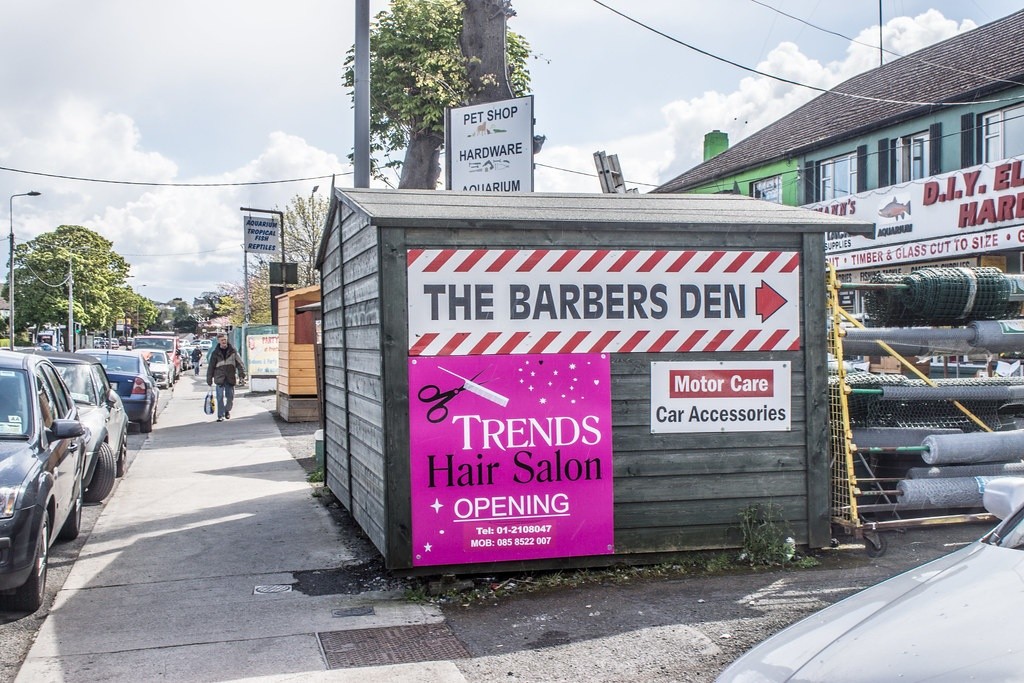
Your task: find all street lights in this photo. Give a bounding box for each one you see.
[135,282,146,336]
[9,190,43,350]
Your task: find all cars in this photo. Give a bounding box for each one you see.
[95,335,133,349]
[178,337,213,371]
[11,350,128,504]
[131,349,177,390]
[0,349,87,614]
[75,346,160,434]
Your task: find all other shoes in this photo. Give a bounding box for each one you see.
[225,411,230,419]
[217,418,223,422]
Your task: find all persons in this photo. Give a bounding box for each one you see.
[192,345,202,375]
[206,332,246,422]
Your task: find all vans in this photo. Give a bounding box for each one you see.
[131,335,182,384]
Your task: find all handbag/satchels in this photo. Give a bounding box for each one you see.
[204,384,216,415]
